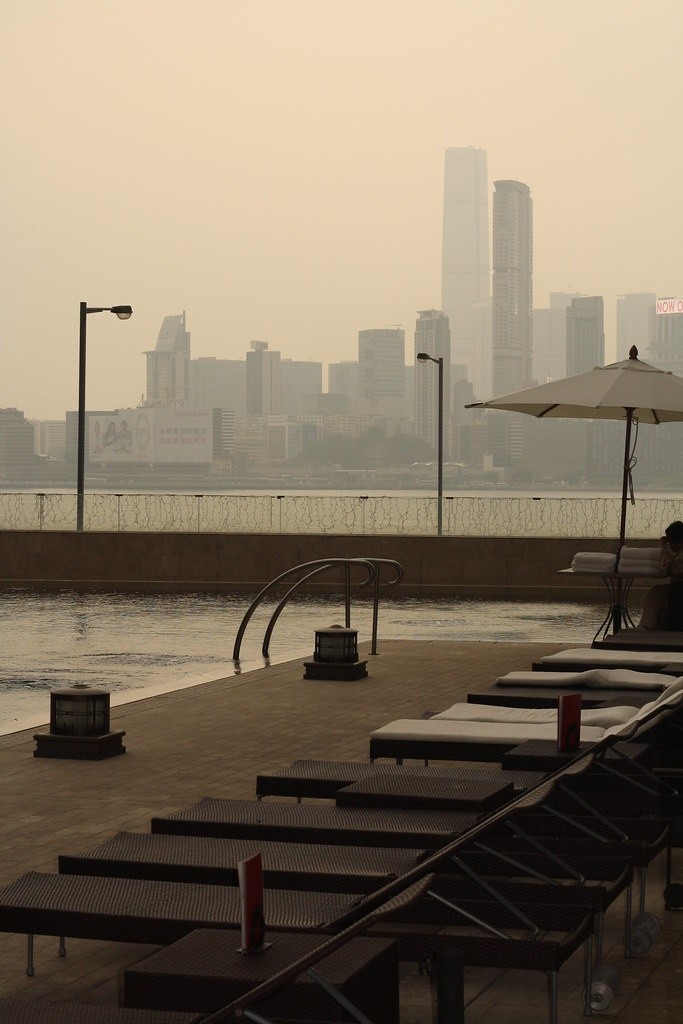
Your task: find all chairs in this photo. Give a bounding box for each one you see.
[420,676,683,729]
[0,780,594,976]
[0,871,434,1024]
[465,669,681,707]
[533,648,683,675]
[59,755,614,992]
[152,720,651,963]
[556,567,666,644]
[256,692,683,911]
[369,690,681,769]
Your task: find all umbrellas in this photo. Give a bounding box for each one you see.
[465,358,683,552]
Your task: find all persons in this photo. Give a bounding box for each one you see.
[640,521,683,629]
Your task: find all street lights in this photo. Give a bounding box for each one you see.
[417,352,442,537]
[77,302,133,532]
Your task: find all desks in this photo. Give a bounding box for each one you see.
[122,926,400,1024]
[660,662,683,675]
[502,739,606,783]
[595,696,657,711]
[336,771,514,813]
